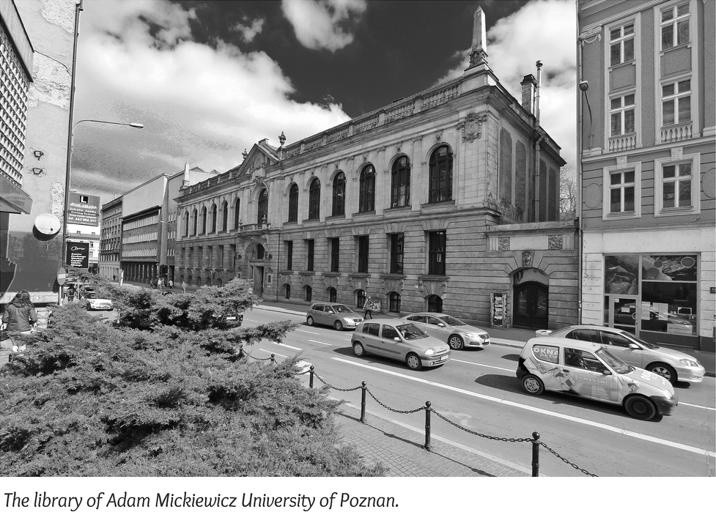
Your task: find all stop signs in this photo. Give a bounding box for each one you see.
[57,268,66,286]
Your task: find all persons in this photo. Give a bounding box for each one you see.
[362,296,373,320]
[157,276,185,294]
[0,288,39,359]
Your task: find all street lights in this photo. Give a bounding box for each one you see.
[59,119,142,267]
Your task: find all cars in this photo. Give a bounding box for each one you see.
[537,324,706,383]
[64,278,114,311]
[408,315,489,350]
[351,319,450,370]
[604,304,692,332]
[517,337,676,420]
[306,303,365,331]
